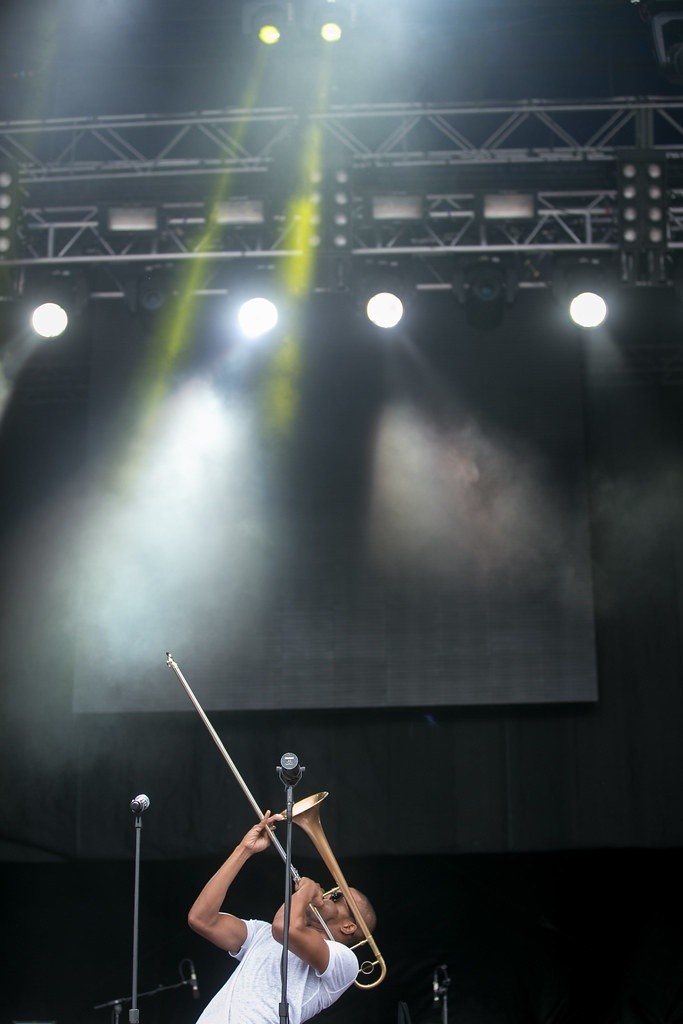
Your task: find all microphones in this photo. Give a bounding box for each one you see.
[431,974,441,1012]
[283,754,298,787]
[132,793,150,816]
[189,970,200,995]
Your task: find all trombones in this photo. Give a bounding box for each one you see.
[162,650,389,991]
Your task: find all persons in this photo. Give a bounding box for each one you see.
[186,809,376,1023]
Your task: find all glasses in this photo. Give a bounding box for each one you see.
[329,892,355,922]
